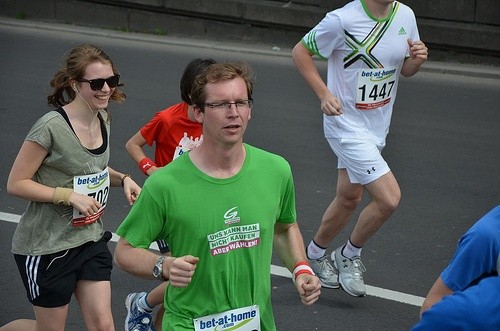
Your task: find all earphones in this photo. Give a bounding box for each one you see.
[72,83,76,86]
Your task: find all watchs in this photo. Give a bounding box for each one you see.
[153,256,169,281]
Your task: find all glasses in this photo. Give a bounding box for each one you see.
[73,73,120,92]
[205,98,253,115]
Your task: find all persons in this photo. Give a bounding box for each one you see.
[291,0,428,296]
[420,204,500,331]
[115,62,321,331]
[0,44,142,331]
[411,277,500,331]
[125,58,217,331]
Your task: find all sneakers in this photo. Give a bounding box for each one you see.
[305,247,341,289]
[331,244,367,298]
[124,291,152,331]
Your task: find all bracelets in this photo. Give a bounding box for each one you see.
[121,174,132,188]
[293,261,314,280]
[137,157,157,175]
[53,187,73,205]
